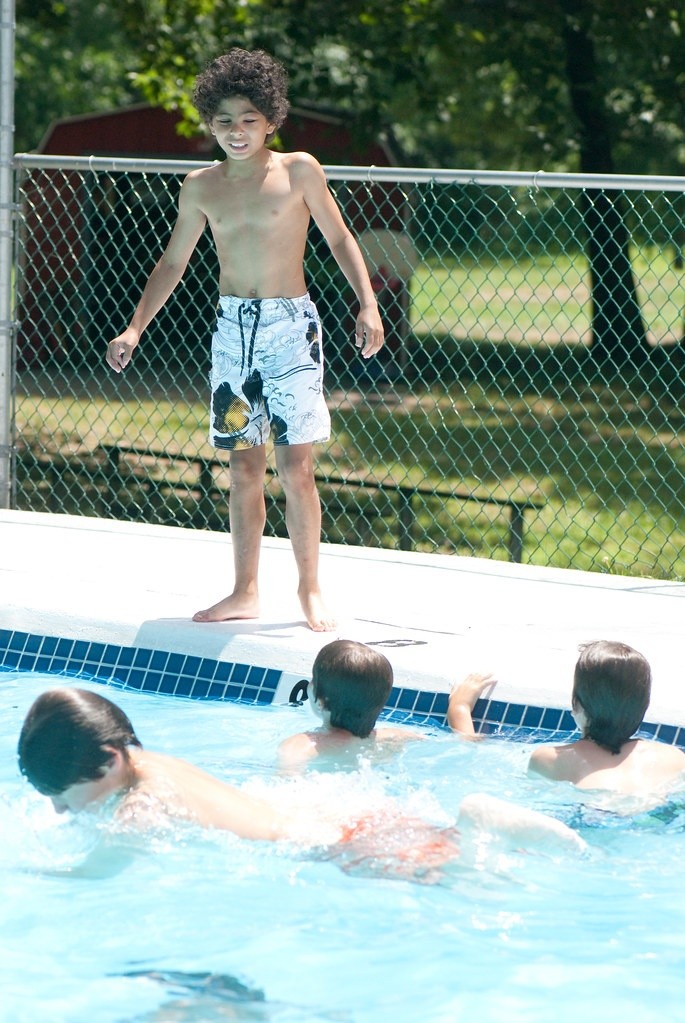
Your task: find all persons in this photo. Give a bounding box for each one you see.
[278,639,498,758]
[105,48,385,632]
[528,641,685,789]
[17,687,293,840]
